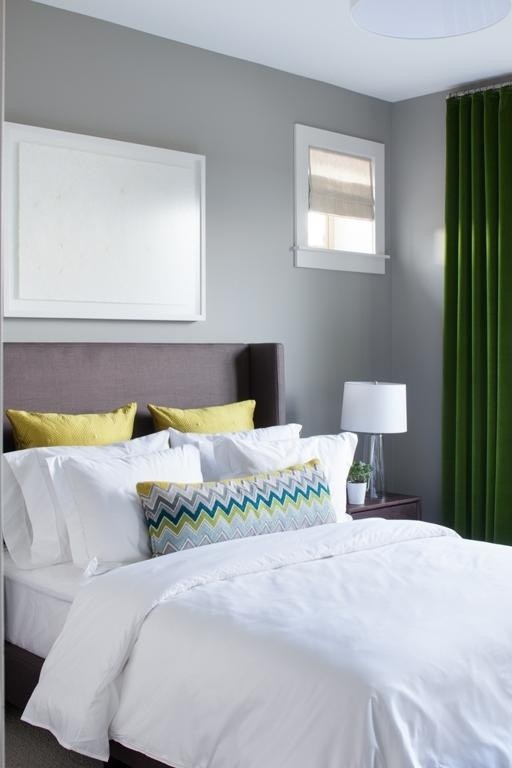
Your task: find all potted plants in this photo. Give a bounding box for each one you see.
[346,461,375,504]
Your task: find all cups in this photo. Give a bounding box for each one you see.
[347,481,367,504]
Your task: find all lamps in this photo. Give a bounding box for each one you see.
[349,1,512,39]
[339,379,409,503]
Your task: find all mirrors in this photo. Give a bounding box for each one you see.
[288,121,391,276]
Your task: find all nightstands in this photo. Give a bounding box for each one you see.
[345,491,421,520]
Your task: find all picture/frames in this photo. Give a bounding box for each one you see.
[2,122,206,321]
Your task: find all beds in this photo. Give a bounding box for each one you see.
[2,341,511,768]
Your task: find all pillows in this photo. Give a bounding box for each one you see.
[46,441,209,576]
[168,422,302,482]
[137,457,336,557]
[4,400,137,449]
[211,430,359,523]
[2,426,172,571]
[146,399,258,434]
